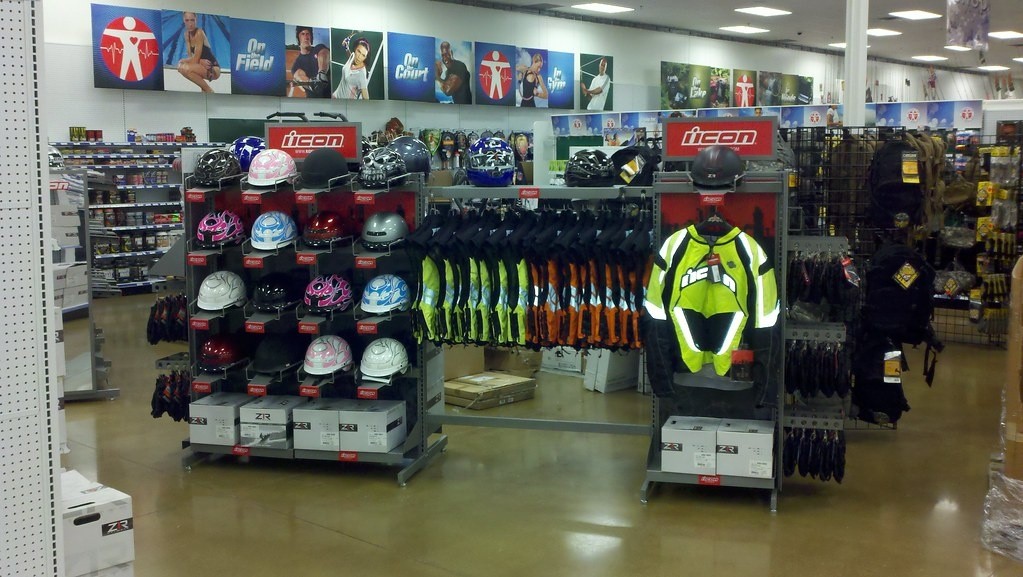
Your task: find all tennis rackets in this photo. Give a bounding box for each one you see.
[341,30,363,47]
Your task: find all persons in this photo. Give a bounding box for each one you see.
[332,30,370,100]
[581,57,610,110]
[826,106,842,126]
[928,66,937,101]
[664,64,688,109]
[605,133,620,145]
[178,12,220,92]
[658,111,696,119]
[516,53,548,107]
[755,108,762,117]
[435,41,472,104]
[291,26,330,98]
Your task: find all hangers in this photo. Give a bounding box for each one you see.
[429,192,652,257]
[695,206,733,232]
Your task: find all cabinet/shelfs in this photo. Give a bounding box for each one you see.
[47,142,448,483]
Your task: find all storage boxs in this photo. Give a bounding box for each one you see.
[661,415,777,479]
[443,371,537,410]
[49,200,406,577]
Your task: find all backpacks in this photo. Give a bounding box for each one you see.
[611,136,660,185]
[844,132,951,423]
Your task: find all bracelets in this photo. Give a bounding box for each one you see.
[535,92,539,96]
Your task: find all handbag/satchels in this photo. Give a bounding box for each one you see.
[932,261,976,293]
[940,222,976,248]
[943,175,977,204]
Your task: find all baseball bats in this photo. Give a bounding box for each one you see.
[285,79,322,88]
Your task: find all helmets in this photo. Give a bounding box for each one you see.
[360,337,408,377]
[564,150,616,187]
[199,333,241,369]
[252,272,299,311]
[357,147,406,189]
[302,210,347,246]
[465,138,515,186]
[362,143,371,156]
[250,210,296,250]
[254,335,301,372]
[693,145,744,185]
[304,335,352,375]
[194,149,241,185]
[197,271,247,310]
[360,274,412,314]
[229,135,265,171]
[386,137,431,178]
[361,212,408,248]
[298,148,348,186]
[196,209,245,246]
[247,149,297,186]
[304,275,353,312]
